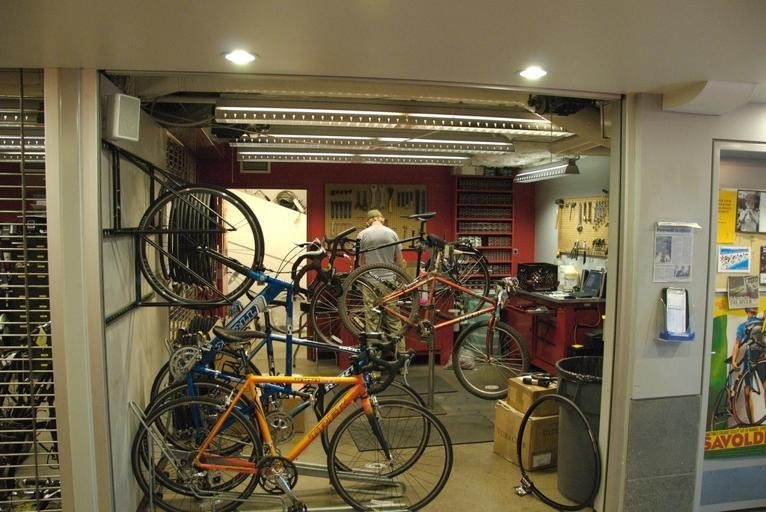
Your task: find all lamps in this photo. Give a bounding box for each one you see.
[566,156,580,174]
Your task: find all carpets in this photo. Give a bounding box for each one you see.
[328,375,493,451]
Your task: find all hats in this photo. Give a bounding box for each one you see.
[368,209,382,220]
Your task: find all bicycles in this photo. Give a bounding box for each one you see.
[708,322,766,432]
[0,314,64,512]
[337,232,531,400]
[134,182,490,511]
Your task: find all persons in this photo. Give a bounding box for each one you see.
[352,207,410,385]
[738,193,760,233]
[731,307,766,425]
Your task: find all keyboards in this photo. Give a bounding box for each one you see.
[569,293,592,298]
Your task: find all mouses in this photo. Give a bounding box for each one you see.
[565,296,576,299]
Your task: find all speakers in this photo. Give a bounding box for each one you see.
[108,94,141,143]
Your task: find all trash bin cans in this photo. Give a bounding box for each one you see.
[555,356,603,504]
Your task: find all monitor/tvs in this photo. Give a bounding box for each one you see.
[582,270,607,296]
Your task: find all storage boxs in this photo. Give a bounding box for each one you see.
[493,371,559,472]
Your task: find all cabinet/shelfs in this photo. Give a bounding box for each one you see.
[454,176,515,324]
[504,292,605,376]
